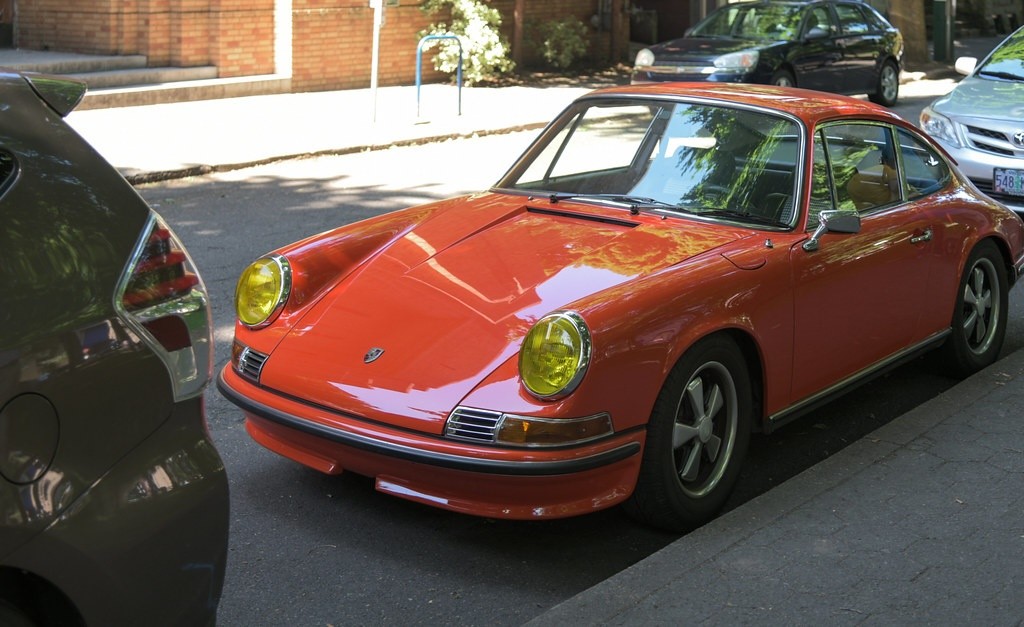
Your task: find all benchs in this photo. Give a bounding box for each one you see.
[730,156,938,207]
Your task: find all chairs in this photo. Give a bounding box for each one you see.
[664,146,860,228]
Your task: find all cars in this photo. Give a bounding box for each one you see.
[0,70,229,626]
[630,0,906,107]
[220,78,1024,526]
[918,25,1024,214]
